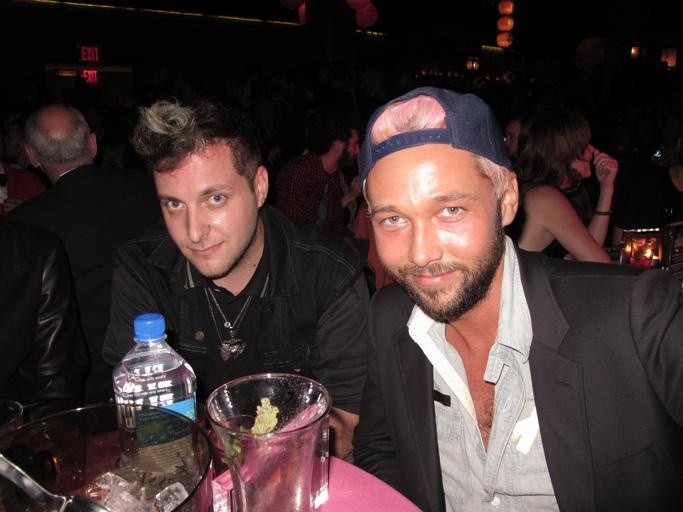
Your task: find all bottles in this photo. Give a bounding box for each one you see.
[109,312,198,430]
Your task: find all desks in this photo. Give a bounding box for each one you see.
[0,431,423,512]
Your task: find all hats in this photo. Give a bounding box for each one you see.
[358,86,522,184]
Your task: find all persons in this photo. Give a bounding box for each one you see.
[1,60,683,512]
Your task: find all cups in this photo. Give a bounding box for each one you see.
[203,372,331,512]
